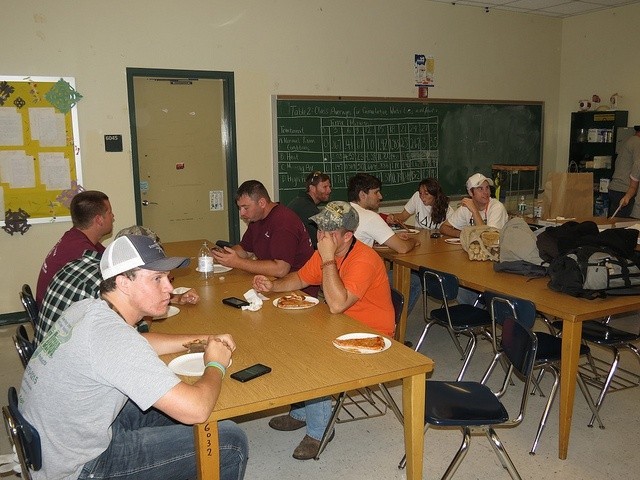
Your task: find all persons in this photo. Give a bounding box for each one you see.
[11,235,249,480]
[607,125,640,219]
[286,172,332,251]
[35,191,115,317]
[611,158,640,219]
[252,200,396,460]
[33,225,199,351]
[440,173,509,310]
[209,180,318,298]
[345,173,422,348]
[386,177,454,229]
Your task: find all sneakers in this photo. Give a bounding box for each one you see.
[269,415,306,431]
[293,429,335,460]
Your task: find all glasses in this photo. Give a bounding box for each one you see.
[312,172,321,185]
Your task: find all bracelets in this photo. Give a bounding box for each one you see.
[321,259,337,269]
[205,362,225,380]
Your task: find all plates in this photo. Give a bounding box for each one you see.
[332,332,393,355]
[195,263,234,274]
[393,228,421,235]
[443,238,462,245]
[151,304,181,320]
[167,351,233,377]
[273,294,320,310]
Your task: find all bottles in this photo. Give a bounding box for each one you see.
[517,195,528,222]
[197,240,215,282]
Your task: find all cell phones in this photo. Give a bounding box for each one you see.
[230,363,271,383]
[212,247,222,253]
[430,233,440,238]
[222,297,249,309]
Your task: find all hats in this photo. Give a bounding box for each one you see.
[114,225,160,242]
[466,173,494,190]
[308,201,359,231]
[100,235,190,281]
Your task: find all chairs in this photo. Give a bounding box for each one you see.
[2,385,43,480]
[18,283,37,327]
[481,289,605,456]
[398,315,540,480]
[530,316,639,429]
[414,265,515,389]
[11,323,33,369]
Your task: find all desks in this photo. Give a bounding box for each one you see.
[394,248,640,461]
[506,204,639,250]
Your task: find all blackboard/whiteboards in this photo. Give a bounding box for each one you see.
[270,94,545,212]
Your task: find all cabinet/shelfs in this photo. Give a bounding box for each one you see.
[567,110,628,214]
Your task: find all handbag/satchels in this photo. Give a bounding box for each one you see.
[536,221,600,264]
[600,227,639,250]
[494,261,548,277]
[541,160,594,222]
[460,226,500,262]
[548,247,640,300]
[500,218,561,268]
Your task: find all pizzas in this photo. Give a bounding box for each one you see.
[332,335,386,353]
[276,292,317,309]
[182,338,208,351]
